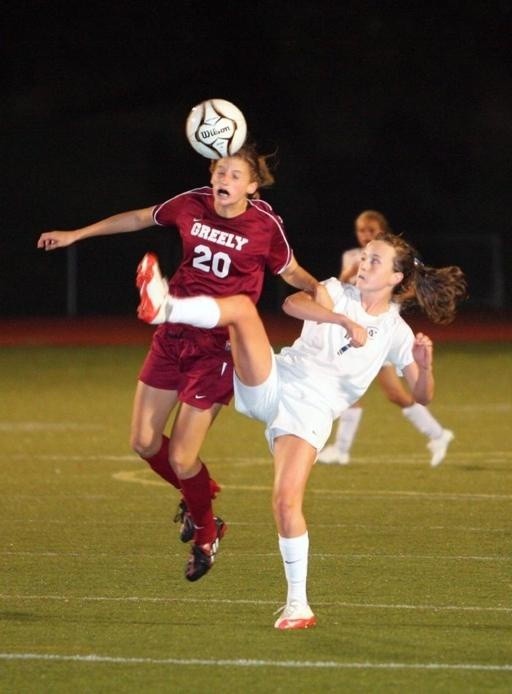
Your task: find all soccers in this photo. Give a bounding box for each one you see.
[186,96,249,162]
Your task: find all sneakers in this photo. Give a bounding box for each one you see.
[273,603,317,631]
[426,429,455,467]
[179,478,228,582]
[135,251,171,326]
[317,444,350,465]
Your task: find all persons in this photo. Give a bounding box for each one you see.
[320,210,455,468]
[134,233,467,632]
[38,146,319,582]
